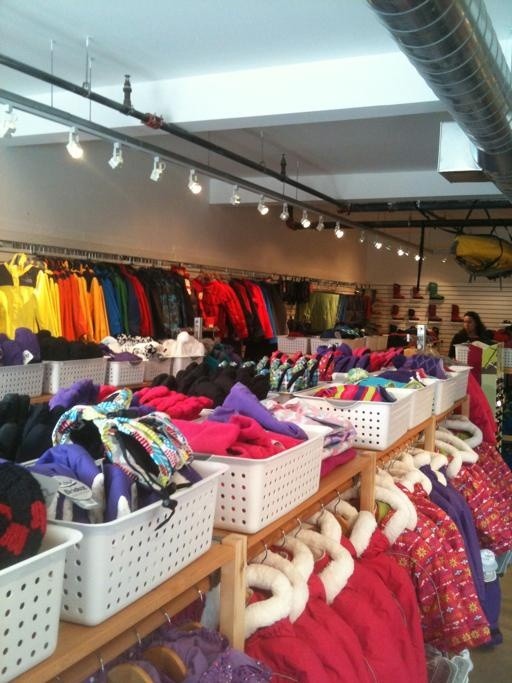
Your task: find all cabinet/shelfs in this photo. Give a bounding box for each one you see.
[481,372,504,453]
[389,296,466,347]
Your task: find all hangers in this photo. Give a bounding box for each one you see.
[250,487,353,575]
[374,434,426,477]
[434,407,457,434]
[12,243,168,282]
[52,584,207,683]
[195,263,256,285]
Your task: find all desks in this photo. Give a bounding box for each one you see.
[435,394,471,435]
[211,453,374,565]
[28,380,152,405]
[1,530,244,683]
[354,416,435,470]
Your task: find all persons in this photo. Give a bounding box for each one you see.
[448,311,493,359]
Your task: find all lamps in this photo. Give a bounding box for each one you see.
[2,97,430,262]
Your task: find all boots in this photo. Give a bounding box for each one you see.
[412,287,423,299]
[428,304,442,321]
[451,304,464,322]
[429,283,444,300]
[408,309,420,320]
[391,305,405,319]
[393,283,405,299]
